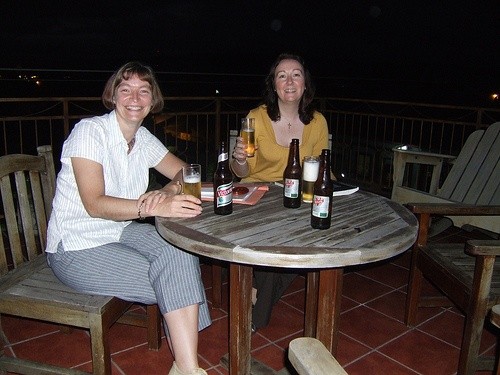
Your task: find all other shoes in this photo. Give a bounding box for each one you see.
[168,362,208,375]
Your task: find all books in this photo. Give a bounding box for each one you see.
[275,175,359,196]
[197,184,257,202]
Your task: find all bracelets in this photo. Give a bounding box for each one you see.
[138,200,145,220]
[171,180,183,195]
[236,159,247,166]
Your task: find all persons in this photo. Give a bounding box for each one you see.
[231,53,329,334]
[46,65,214,375]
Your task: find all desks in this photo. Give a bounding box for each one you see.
[155,182,419,375]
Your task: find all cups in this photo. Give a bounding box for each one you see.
[303,156,319,203]
[241,118,255,158]
[182,164,202,205]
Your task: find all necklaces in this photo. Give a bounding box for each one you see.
[281,115,296,128]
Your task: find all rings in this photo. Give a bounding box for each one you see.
[157,193,162,197]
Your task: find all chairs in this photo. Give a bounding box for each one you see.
[0,145,162,375]
[392,122,500,375]
[211,130,333,338]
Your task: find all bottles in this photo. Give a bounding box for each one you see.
[282,138,302,209]
[214,140,233,216]
[312,148,333,230]
